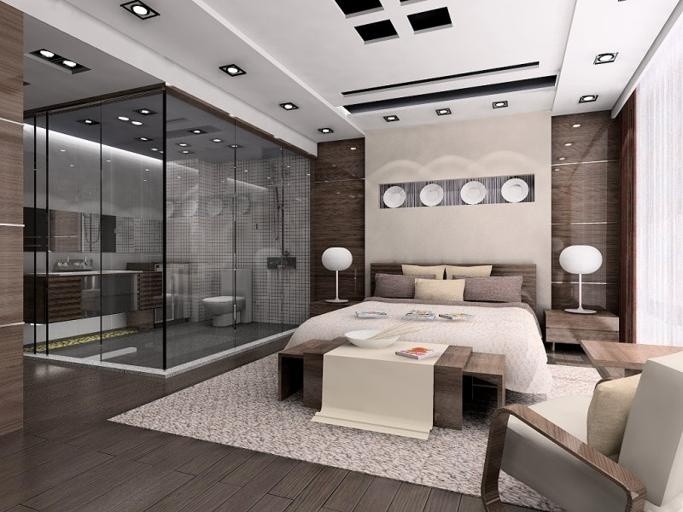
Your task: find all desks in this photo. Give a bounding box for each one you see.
[579,340,682,379]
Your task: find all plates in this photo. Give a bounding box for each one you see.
[346,329,399,348]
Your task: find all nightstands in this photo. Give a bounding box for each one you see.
[543,305,620,352]
[309,302,358,317]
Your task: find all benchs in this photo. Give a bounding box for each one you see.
[278,337,506,430]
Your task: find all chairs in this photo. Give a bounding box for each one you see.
[481,350,682,511]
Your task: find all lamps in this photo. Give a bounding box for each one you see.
[559,244,603,314]
[322,246,354,302]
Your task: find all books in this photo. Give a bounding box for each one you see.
[439,313,472,318]
[403,310,436,320]
[356,310,387,319]
[395,347,434,359]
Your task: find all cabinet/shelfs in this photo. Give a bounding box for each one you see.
[23,206,163,254]
[127,262,191,332]
[22,271,161,324]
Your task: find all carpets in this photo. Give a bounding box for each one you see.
[23,326,141,353]
[106,350,604,511]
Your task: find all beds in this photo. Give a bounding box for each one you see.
[282,263,552,395]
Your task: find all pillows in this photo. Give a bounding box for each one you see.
[586,372,643,457]
[415,278,465,302]
[401,264,446,279]
[465,277,524,302]
[446,265,493,281]
[375,273,435,299]
